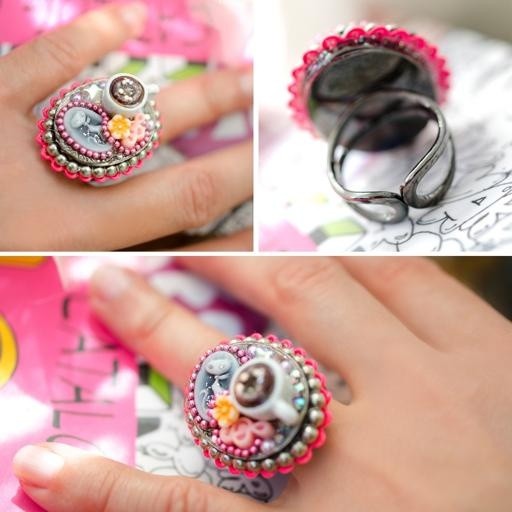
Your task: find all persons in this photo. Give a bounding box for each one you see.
[0,0,253,251]
[10,255,512,511]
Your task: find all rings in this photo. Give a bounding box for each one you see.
[35,70,166,185]
[285,17,457,224]
[183,331,333,478]
